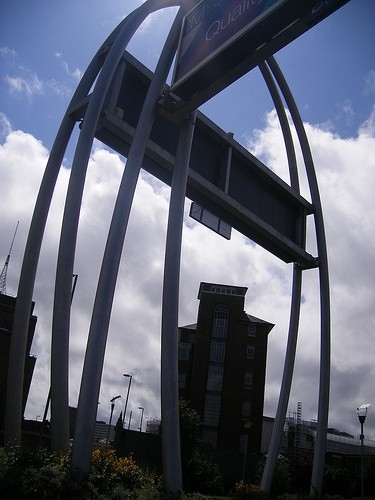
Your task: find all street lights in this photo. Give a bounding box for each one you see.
[104,395,122,454]
[123,373,132,429]
[138,406,145,432]
